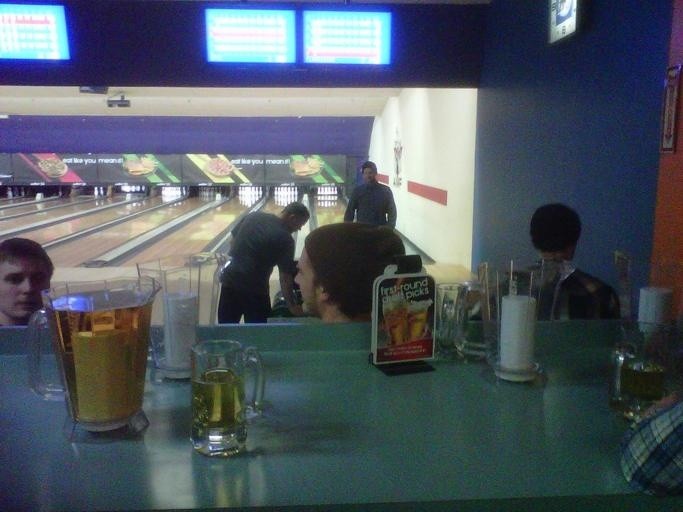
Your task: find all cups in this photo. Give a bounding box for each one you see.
[185,339,266,460]
[435,284,467,360]
[621,319,669,421]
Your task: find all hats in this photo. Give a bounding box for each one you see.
[361,162,377,171]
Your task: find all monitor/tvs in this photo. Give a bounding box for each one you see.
[1,0,76,70]
[299,7,397,76]
[199,3,299,83]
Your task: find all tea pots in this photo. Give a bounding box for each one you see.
[26,252,232,445]
[453,258,577,384]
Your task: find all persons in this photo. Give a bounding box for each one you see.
[217,201,310,324]
[511,201,621,320]
[343,161,398,231]
[1,238,54,326]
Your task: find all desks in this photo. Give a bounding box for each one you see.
[1,317,683,511]
